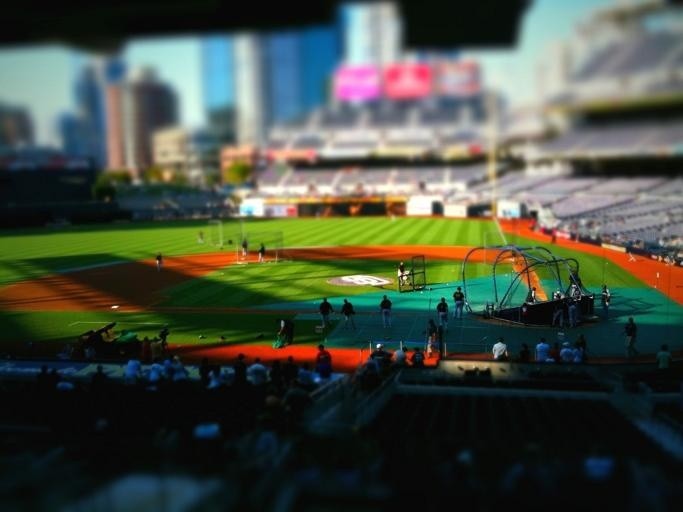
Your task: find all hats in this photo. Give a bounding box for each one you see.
[376,344,382,349]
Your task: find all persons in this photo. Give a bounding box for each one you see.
[1,169,683,512]
